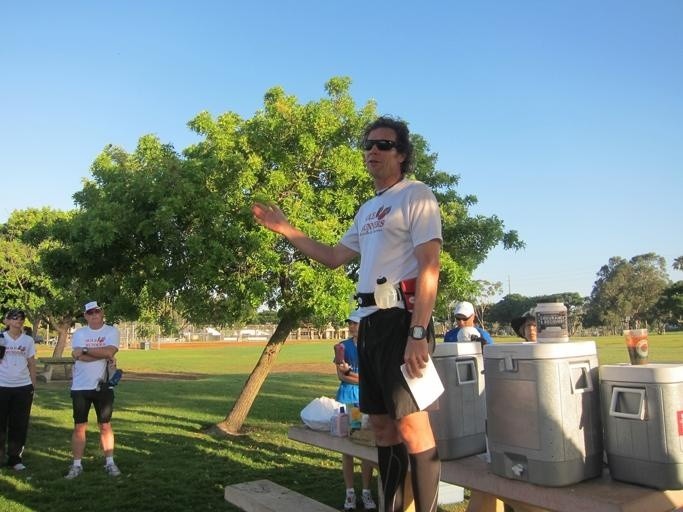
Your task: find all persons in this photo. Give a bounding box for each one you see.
[0,308,40,474]
[333,313,378,512]
[252,117,445,512]
[511,307,538,343]
[0,308,33,336]
[64,301,123,480]
[442,302,493,353]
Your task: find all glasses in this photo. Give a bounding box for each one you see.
[456,315,473,321]
[8,315,25,320]
[363,140,400,150]
[86,309,102,315]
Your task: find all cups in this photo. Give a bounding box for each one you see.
[623,329,650,366]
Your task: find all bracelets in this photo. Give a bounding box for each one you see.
[344,368,353,375]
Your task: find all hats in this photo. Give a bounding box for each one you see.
[511,311,537,336]
[9,308,24,317]
[84,301,102,313]
[457,327,480,342]
[454,301,474,317]
[344,311,361,323]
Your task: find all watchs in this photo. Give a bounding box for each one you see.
[82,346,88,356]
[408,324,429,341]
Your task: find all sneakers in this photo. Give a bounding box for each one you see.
[361,489,376,509]
[64,465,83,479]
[12,463,26,470]
[344,489,357,510]
[104,464,121,477]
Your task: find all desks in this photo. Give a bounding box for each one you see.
[288,421,681,512]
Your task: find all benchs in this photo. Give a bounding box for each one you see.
[221,479,339,512]
